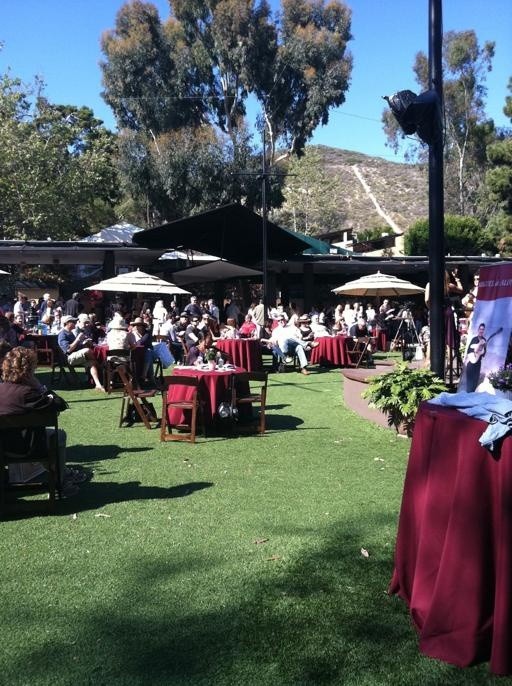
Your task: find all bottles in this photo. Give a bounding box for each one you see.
[130,318,148,325]
[62,315,78,323]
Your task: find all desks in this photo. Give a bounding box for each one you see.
[162,365,249,432]
[387,398,512,676]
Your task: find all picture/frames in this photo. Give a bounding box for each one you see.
[387,398,512,676]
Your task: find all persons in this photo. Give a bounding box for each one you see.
[184,328,229,365]
[0,293,481,382]
[350,320,369,338]
[464,320,489,395]
[0,346,94,488]
[268,314,320,375]
[425,269,464,371]
[462,270,480,310]
[58,315,106,393]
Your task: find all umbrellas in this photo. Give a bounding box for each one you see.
[311,342,319,348]
[301,369,310,375]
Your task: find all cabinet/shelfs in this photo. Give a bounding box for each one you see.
[216,352,224,371]
[197,356,202,368]
[38,321,51,335]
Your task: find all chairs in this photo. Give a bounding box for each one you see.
[159,375,207,444]
[116,363,158,430]
[1,411,63,509]
[238,371,270,432]
[0,324,369,392]
